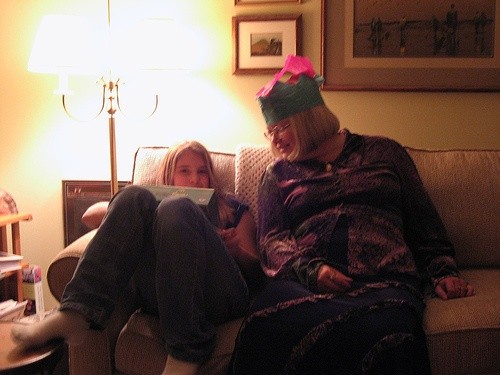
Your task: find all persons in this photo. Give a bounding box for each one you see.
[10,141,259,375]
[225,76,475,375]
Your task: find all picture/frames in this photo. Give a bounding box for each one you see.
[320,0,500,93]
[232,13,304,75]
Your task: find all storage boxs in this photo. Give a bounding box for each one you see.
[24,263,45,317]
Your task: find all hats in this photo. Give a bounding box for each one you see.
[254,53,325,127]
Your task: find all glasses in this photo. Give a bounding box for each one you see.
[264,123,290,139]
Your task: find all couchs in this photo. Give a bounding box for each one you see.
[47,146,500,375]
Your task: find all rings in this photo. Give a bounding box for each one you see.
[330,272,336,280]
[454,285,461,288]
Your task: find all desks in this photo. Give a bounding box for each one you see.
[0,211,33,302]
[0,321,70,375]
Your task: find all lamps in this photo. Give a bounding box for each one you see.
[28,0,160,197]
